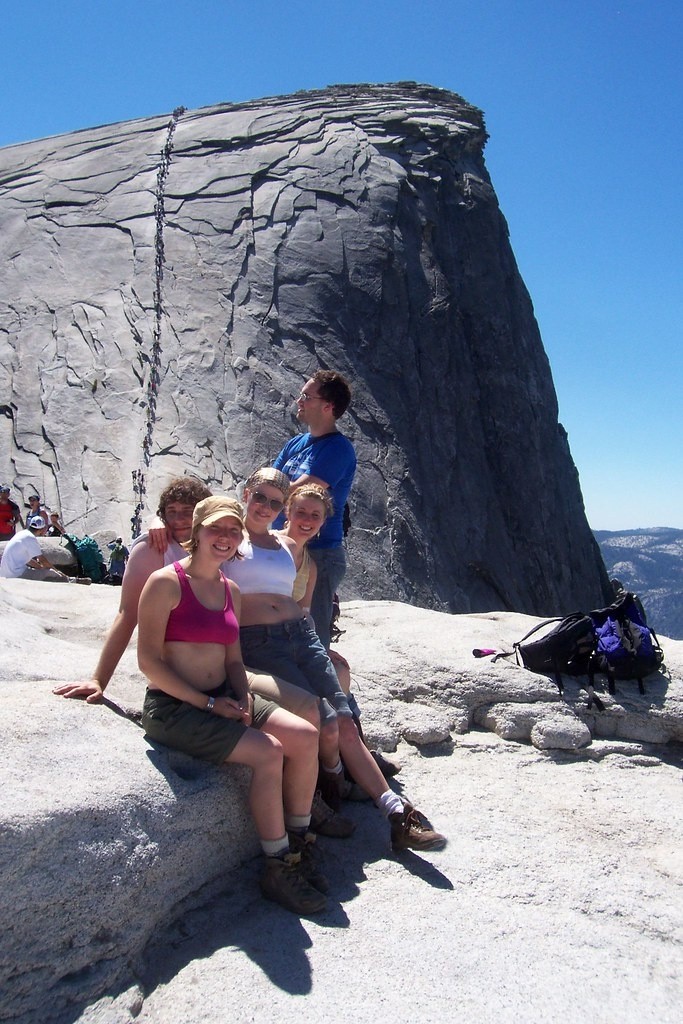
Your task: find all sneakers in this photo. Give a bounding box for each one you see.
[369,747,402,779]
[287,830,329,895]
[387,803,449,855]
[259,850,326,914]
[322,766,372,803]
[311,790,358,839]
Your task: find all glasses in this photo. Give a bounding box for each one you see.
[298,392,324,401]
[248,490,285,514]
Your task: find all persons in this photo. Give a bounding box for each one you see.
[149,468,445,851]
[26,495,49,537]
[136,495,331,915]
[47,513,66,537]
[266,483,401,802]
[0,486,21,541]
[106,538,129,575]
[52,478,355,838]
[273,369,357,651]
[0,516,91,586]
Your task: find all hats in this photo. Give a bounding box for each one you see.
[30,515,45,530]
[115,537,123,543]
[191,495,245,529]
[0,486,10,493]
[29,494,41,502]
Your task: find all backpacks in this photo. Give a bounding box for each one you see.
[111,543,125,561]
[513,611,607,683]
[588,592,664,681]
[68,534,109,582]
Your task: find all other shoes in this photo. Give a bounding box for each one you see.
[76,576,91,585]
